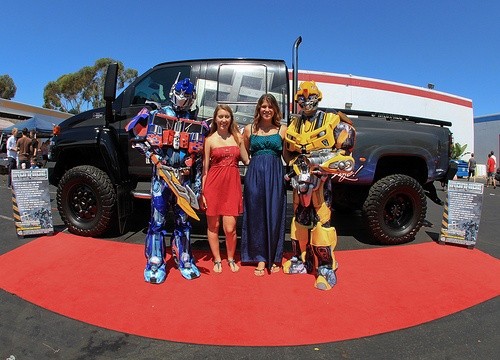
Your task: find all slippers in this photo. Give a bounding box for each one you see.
[228,260,240,272]
[270,264,280,272]
[254,268,265,276]
[213,261,222,273]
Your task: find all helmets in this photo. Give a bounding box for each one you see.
[168,72,197,111]
[294,80,322,111]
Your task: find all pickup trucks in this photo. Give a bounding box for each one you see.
[43,36,458,246]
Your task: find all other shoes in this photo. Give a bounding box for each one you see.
[485,185,489,187]
[178,258,200,280]
[493,186,496,189]
[144,261,166,285]
[7,187,12,189]
[282,256,313,274]
[312,265,337,290]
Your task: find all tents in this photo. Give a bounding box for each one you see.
[0,114,66,156]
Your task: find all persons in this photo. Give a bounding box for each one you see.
[241,94,288,276]
[199,104,250,272]
[124,71,210,285]
[441,151,497,191]
[281,80,357,290]
[6,127,51,189]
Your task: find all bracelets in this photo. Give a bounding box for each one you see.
[199,193,204,194]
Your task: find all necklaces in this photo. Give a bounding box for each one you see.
[260,126,268,130]
